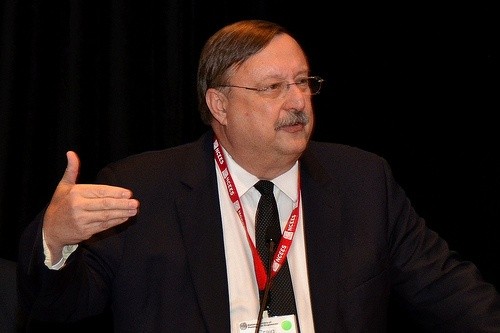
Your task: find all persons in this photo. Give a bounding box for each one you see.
[0,20,500,333]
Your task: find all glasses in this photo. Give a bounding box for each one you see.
[216,77,324,101]
[253,179,301,333]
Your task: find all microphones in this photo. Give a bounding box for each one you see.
[255,225,279,333]
